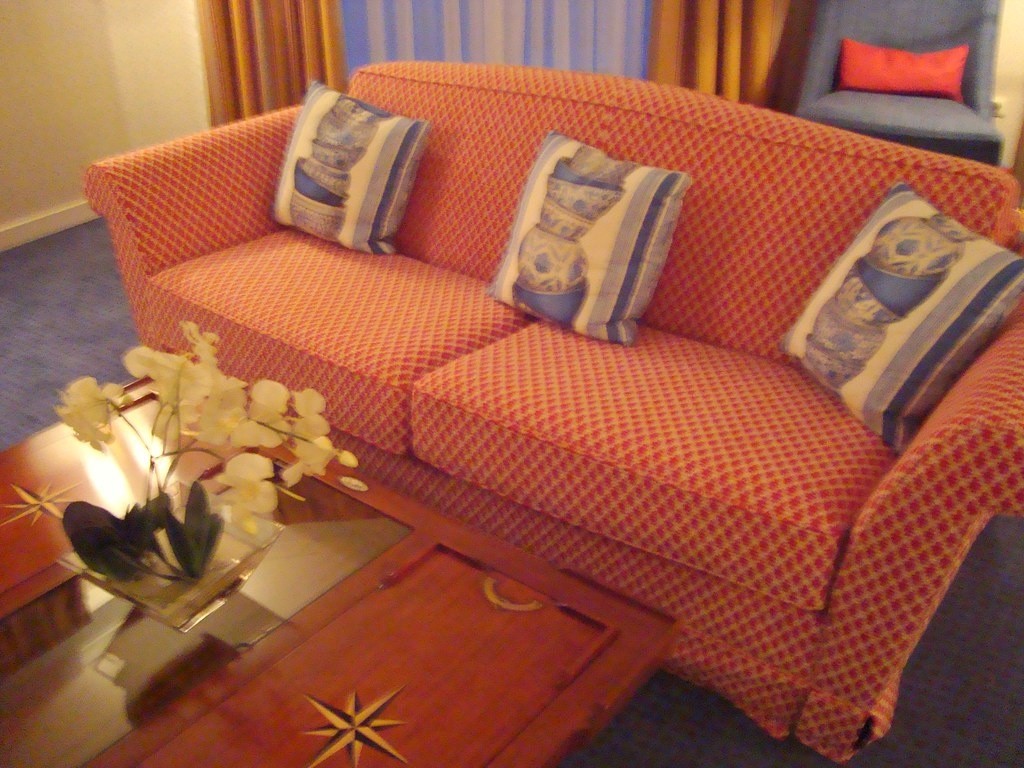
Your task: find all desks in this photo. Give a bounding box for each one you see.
[0,357,680,768]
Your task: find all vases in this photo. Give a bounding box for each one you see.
[53,478,287,634]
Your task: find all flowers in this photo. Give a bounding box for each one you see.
[53,321,359,586]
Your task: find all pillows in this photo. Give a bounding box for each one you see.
[780,183,1024,454]
[837,37,969,106]
[486,127,695,347]
[270,78,433,254]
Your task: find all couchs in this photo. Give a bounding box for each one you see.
[794,0,1004,167]
[82,61,1024,762]
